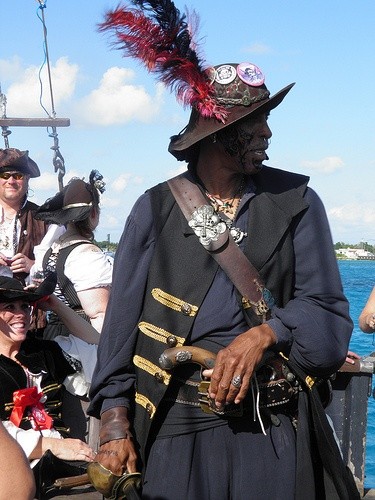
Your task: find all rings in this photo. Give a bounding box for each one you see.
[231,375,242,389]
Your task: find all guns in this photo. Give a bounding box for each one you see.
[160,346,222,381]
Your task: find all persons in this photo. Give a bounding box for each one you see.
[0,148,115,500]
[358,284,375,334]
[86,0,361,500]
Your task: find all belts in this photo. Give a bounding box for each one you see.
[172,379,293,410]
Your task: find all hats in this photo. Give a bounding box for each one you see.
[0,272,57,302]
[31,168,106,225]
[0,148,41,178]
[168,63,296,161]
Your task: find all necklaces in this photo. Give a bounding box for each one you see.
[205,182,249,248]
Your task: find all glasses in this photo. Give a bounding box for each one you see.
[0,173,30,180]
